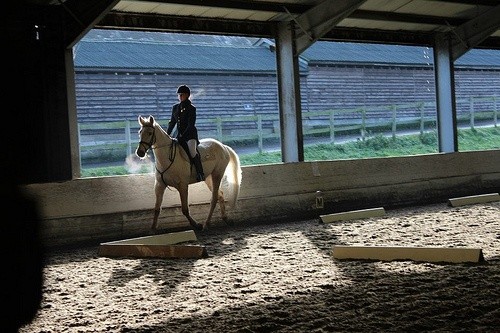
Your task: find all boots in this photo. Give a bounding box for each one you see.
[193,153,204,181]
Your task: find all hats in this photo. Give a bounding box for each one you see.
[177,85,190,93]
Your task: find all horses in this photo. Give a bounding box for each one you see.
[135,114,243,231]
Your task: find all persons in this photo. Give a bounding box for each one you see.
[166,84,204,182]
